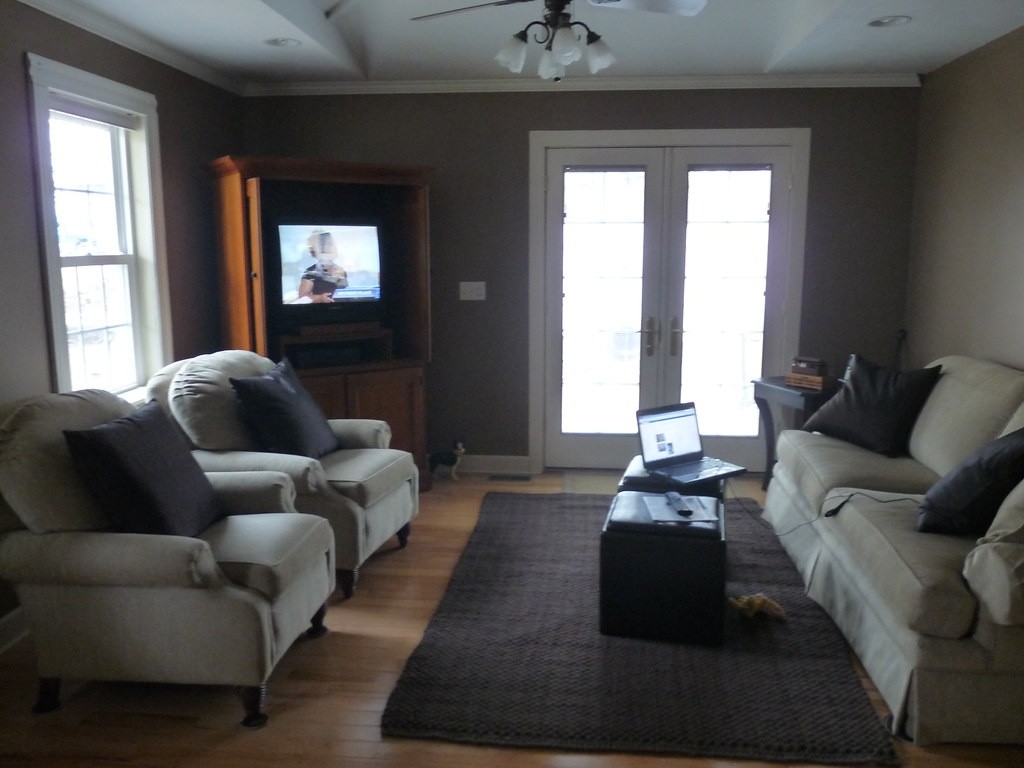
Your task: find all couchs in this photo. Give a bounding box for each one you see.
[0,388,336,730]
[759,358,1024,750]
[144,348,419,600]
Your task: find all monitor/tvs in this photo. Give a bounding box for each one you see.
[259,210,400,326]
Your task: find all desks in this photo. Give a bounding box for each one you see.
[598,491,728,649]
[617,453,727,503]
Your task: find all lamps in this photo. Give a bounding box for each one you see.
[494,10,617,80]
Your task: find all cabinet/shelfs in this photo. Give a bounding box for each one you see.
[200,153,438,493]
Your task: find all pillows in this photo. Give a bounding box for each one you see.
[61,396,232,538]
[913,426,1024,541]
[227,356,342,459]
[801,352,943,458]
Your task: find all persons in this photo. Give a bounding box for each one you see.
[298,232,350,303]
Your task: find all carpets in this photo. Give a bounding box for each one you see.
[380,491,905,768]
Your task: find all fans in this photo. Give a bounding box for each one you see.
[410,0,709,23]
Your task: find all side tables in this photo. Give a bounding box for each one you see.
[750,375,839,493]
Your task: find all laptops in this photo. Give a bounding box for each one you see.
[635,402,747,488]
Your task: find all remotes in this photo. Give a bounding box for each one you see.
[665,492,694,517]
